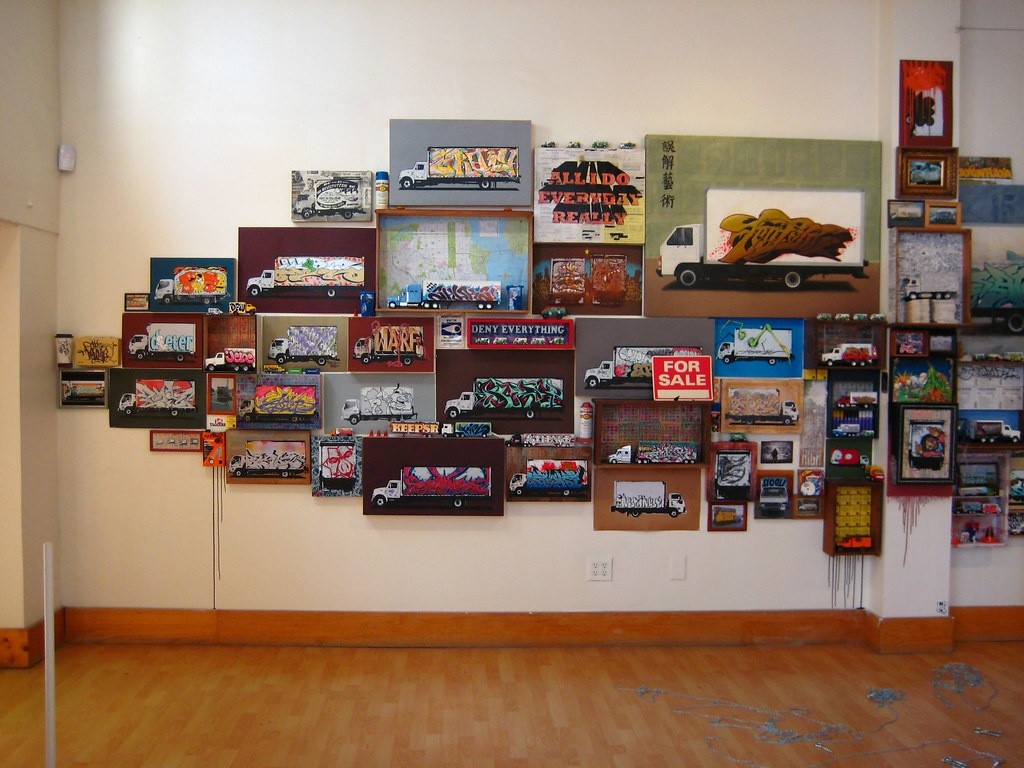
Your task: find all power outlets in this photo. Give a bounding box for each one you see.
[586,557,613,581]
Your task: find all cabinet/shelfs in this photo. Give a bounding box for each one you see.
[824,477,882,557]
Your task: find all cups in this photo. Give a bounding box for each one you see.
[55,333,74,368]
[506,284,523,310]
[361,290,375,317]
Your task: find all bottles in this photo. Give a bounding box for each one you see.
[375,172,388,210]
[579,402,593,438]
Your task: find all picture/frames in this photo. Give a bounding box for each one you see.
[924,201,961,230]
[896,402,958,484]
[896,146,959,200]
[706,442,758,503]
[792,494,823,519]
[708,500,747,532]
